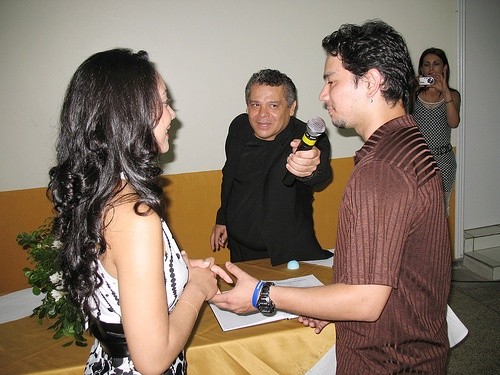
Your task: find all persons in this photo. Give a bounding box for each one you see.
[416,47,460,217]
[208,23,453,374]
[47,49,233,375]
[210,70,333,266]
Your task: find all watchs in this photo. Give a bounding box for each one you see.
[257,282,277,317]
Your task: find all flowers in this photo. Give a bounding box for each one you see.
[16,226,89,347]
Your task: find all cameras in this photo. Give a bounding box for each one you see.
[419,77,435,85]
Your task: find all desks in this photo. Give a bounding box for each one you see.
[0,258,336,375]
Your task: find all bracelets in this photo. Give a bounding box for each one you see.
[444,99,453,104]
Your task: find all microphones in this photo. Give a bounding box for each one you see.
[281,117,326,188]
